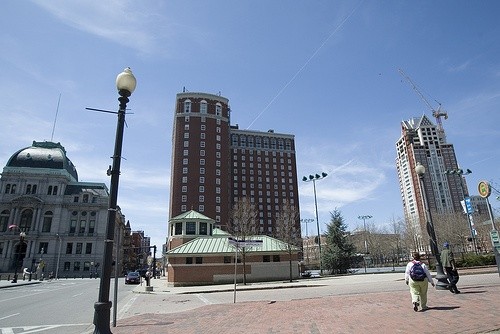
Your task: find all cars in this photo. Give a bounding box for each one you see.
[124,272,140,285]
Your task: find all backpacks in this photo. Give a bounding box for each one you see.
[410,260,425,281]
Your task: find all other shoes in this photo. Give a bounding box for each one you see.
[413,302,418,311]
[422,306,428,311]
[454,290,460,294]
[446,285,454,293]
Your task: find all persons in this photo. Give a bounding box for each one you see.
[143,268,151,286]
[405,252,435,312]
[440,243,460,294]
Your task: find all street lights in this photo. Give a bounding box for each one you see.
[414,161,450,290]
[12,231,26,283]
[302,172,328,275]
[91,66,137,334]
[444,168,479,255]
[357,215,373,231]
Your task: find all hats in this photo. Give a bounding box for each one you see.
[443,243,449,247]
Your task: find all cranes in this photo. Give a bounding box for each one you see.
[395,64,448,132]
